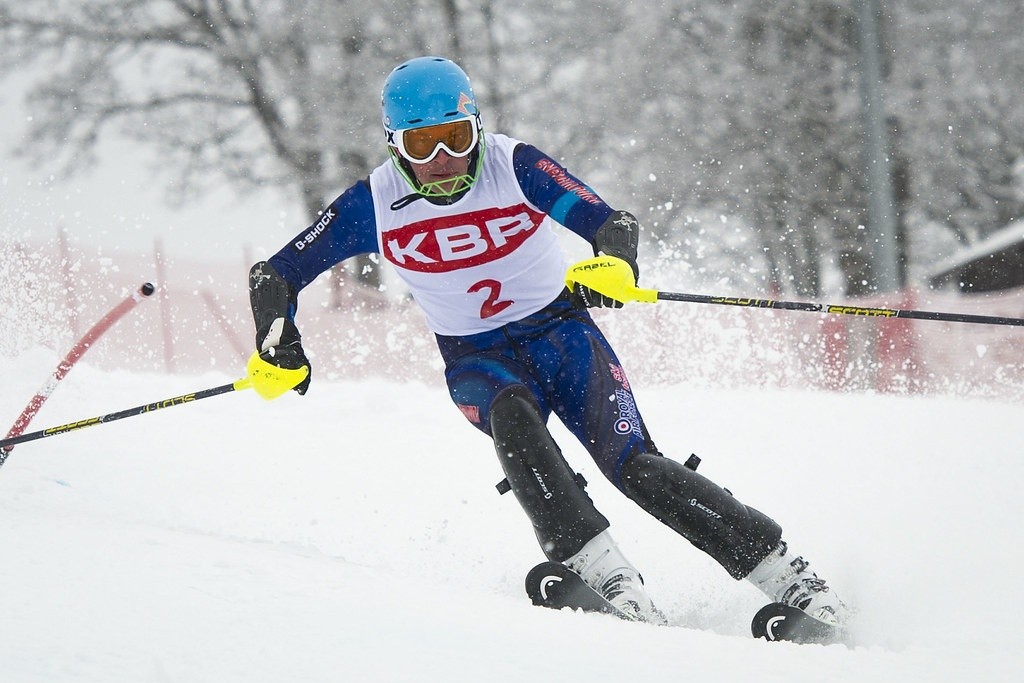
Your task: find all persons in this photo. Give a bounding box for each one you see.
[249,55,855,644]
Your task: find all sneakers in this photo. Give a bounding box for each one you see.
[563,530,657,622]
[744,542,847,624]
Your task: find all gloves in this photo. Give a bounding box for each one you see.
[573,210,640,309]
[248,260,312,395]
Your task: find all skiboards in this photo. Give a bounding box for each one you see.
[526,561,857,647]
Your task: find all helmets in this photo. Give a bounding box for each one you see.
[381,56,486,148]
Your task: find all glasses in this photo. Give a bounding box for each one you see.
[382,111,483,165]
